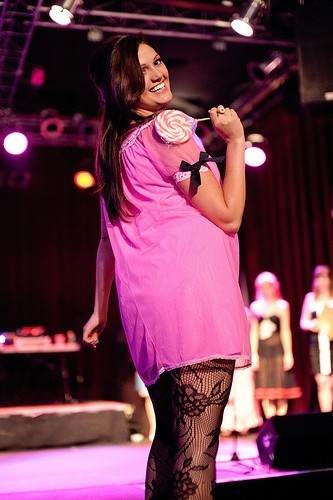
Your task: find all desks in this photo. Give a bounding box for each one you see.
[0,342,80,404]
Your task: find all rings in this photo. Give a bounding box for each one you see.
[217,108,225,114]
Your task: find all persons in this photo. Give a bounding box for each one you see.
[82,34,246,500]
[222,307,263,437]
[300,266,333,412]
[250,271,302,419]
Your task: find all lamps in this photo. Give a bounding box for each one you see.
[231,0,267,36]
[40,109,64,139]
[71,114,95,138]
[49,0,83,25]
